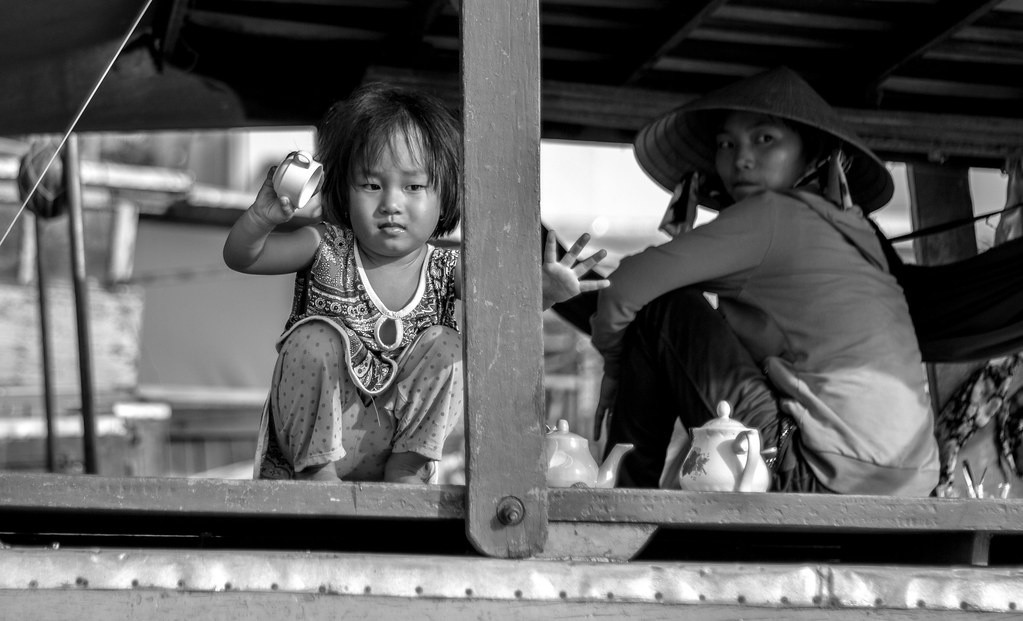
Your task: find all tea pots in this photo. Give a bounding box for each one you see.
[545,418,634,487]
[678,401,769,493]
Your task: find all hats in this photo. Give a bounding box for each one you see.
[634,61,894,215]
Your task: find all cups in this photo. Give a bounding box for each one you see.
[271,150,324,209]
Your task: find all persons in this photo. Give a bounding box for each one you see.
[223,85,610,483]
[591,67,941,496]
